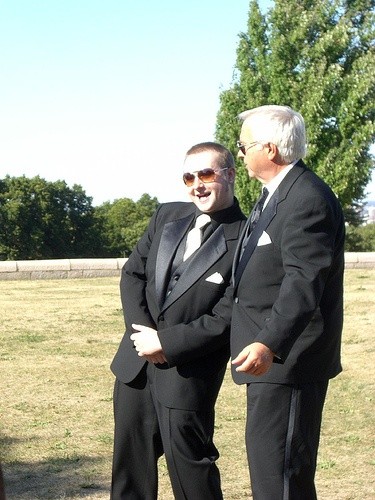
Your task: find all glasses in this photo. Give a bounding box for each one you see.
[182,167,230,186]
[236,139,268,153]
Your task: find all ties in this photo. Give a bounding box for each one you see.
[182,214,210,261]
[247,187,269,237]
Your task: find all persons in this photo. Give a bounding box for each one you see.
[110,142,248,500]
[230,105,346,500]
